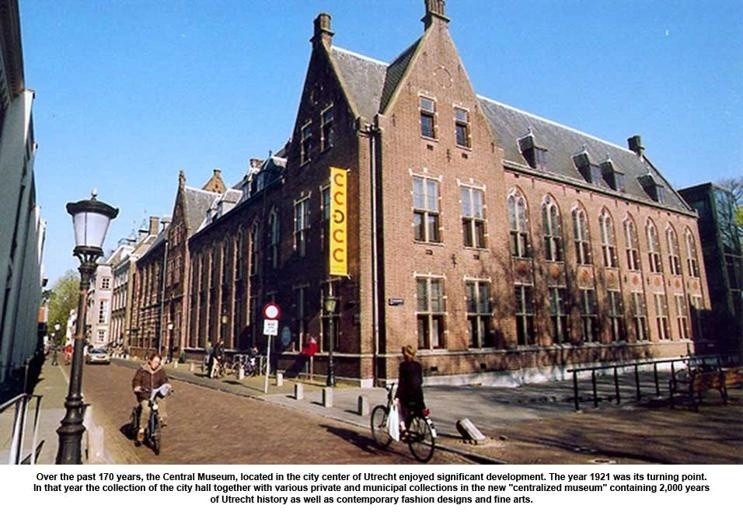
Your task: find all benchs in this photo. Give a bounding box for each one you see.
[669,366,743,413]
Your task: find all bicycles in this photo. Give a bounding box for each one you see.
[370,381,437,462]
[131,386,175,456]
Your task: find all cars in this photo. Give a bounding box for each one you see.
[85,347,112,366]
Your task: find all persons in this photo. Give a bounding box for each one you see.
[50,350,58,365]
[284,333,319,380]
[207,339,226,378]
[389,345,425,442]
[126,352,171,445]
[62,344,74,364]
[239,326,260,368]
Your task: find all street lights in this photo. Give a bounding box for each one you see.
[51,320,61,366]
[220,312,230,367]
[323,294,339,388]
[56,192,120,464]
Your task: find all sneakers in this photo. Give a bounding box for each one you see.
[137,429,145,441]
[162,423,167,427]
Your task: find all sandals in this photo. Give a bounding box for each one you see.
[400,430,408,440]
